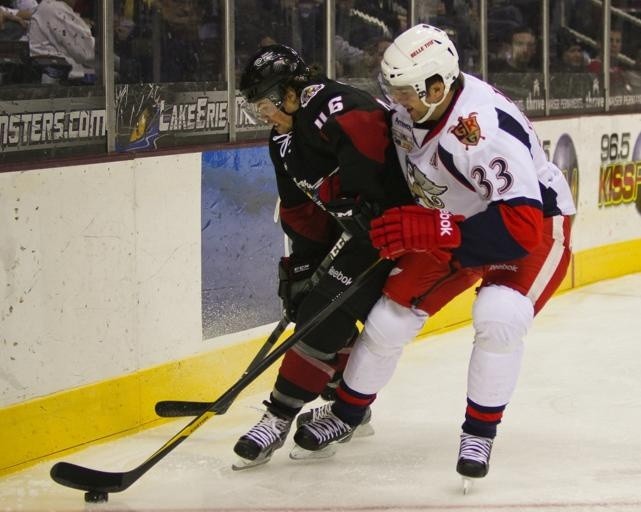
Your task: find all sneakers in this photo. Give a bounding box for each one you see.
[232,400,294,461]
[292,399,372,451]
[456,421,495,478]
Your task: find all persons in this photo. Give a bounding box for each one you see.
[0,0,635,101]
[294,23,576,477]
[233,43,417,460]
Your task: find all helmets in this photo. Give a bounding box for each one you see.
[239,44,311,108]
[381,22,461,108]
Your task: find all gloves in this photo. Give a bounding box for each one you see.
[278,257,327,323]
[368,203,466,263]
[328,181,387,238]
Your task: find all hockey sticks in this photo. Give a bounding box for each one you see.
[50,257,385,493]
[154,231,352,417]
